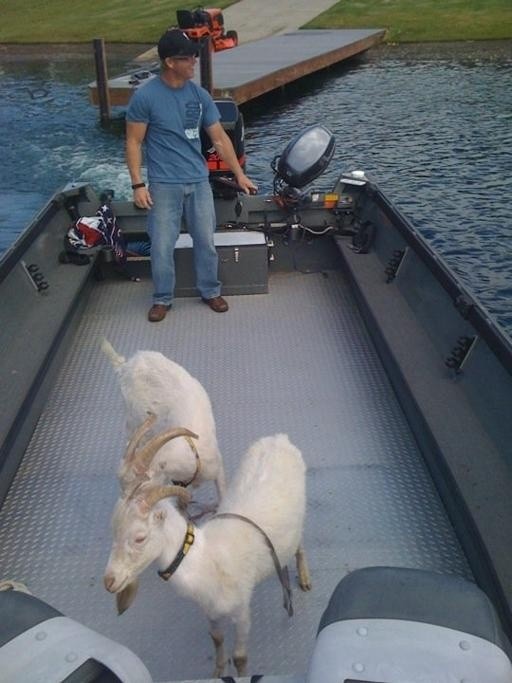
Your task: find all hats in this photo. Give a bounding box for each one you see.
[158,31,205,59]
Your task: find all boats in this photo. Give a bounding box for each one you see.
[0,95,512,679]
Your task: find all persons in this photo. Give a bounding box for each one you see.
[125,30,258,321]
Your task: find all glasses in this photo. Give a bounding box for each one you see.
[171,55,195,62]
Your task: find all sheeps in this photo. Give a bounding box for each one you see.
[100,338,228,502]
[103,432,311,680]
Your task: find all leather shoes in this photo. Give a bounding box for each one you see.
[148,304,171,322]
[202,296,228,312]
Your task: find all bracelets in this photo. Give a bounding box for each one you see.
[132,183,145,189]
